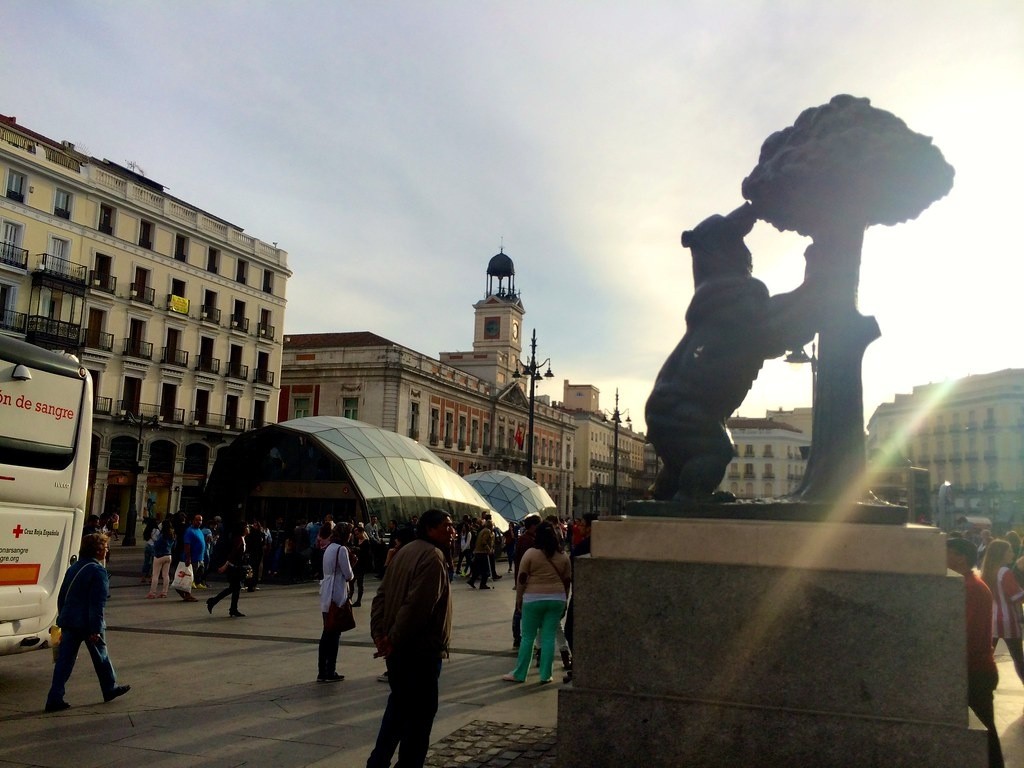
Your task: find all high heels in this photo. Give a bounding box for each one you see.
[228,611,246,618]
[206,598,214,614]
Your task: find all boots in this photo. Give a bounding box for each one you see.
[561,651,572,669]
[537,648,541,666]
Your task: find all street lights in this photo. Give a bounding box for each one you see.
[510,328,554,480]
[118,412,165,545]
[784,343,818,430]
[601,387,631,516]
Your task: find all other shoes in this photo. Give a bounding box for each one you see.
[378,673,389,682]
[541,677,553,684]
[196,584,207,589]
[183,597,198,602]
[351,601,361,607]
[316,673,344,683]
[449,569,513,591]
[191,582,197,588]
[156,593,167,598]
[502,673,525,682]
[114,538,119,541]
[104,685,132,703]
[45,701,70,712]
[145,594,155,599]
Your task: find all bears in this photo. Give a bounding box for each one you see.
[644,202,825,501]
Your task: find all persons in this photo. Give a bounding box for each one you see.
[454,512,599,685]
[366,508,455,768]
[44,535,131,714]
[945,528,1024,768]
[142,514,419,618]
[84,510,120,541]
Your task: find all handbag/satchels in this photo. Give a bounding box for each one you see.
[51,625,63,663]
[326,600,356,632]
[170,561,193,593]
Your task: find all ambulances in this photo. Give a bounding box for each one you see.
[0,329,96,663]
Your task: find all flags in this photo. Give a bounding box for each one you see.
[515,426,526,451]
